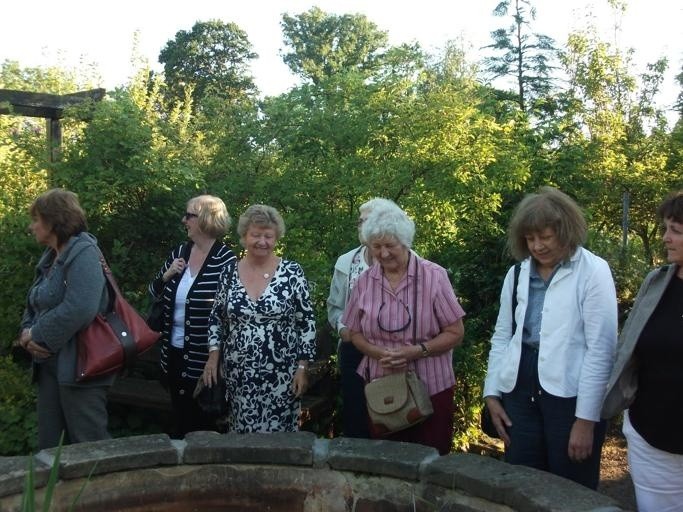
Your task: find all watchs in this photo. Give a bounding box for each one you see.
[419,344,428,357]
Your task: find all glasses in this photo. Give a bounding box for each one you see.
[186,212,198,220]
[377,302,411,332]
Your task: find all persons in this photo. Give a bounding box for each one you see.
[20,188,114,451]
[203,204,317,436]
[482,192,619,491]
[600,194,683,512]
[148,194,237,440]
[327,200,398,438]
[340,206,467,456]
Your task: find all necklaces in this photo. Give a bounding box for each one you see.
[249,263,273,280]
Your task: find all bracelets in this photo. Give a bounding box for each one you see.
[29,327,33,339]
[298,365,309,372]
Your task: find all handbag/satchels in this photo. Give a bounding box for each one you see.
[76,297,163,381]
[481,404,501,438]
[144,295,163,331]
[364,370,434,436]
[193,370,227,417]
